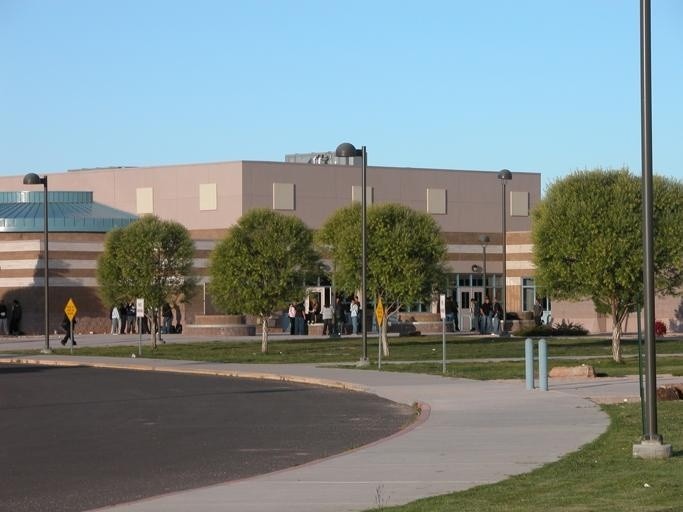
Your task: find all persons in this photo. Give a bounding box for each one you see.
[447,296,503,336]
[61,313,76,345]
[533,299,543,327]
[0,300,22,336]
[109,302,174,335]
[288,297,361,336]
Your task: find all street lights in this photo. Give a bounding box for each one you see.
[480,233,490,306]
[496,167,515,327]
[335,140,369,368]
[22,171,50,353]
